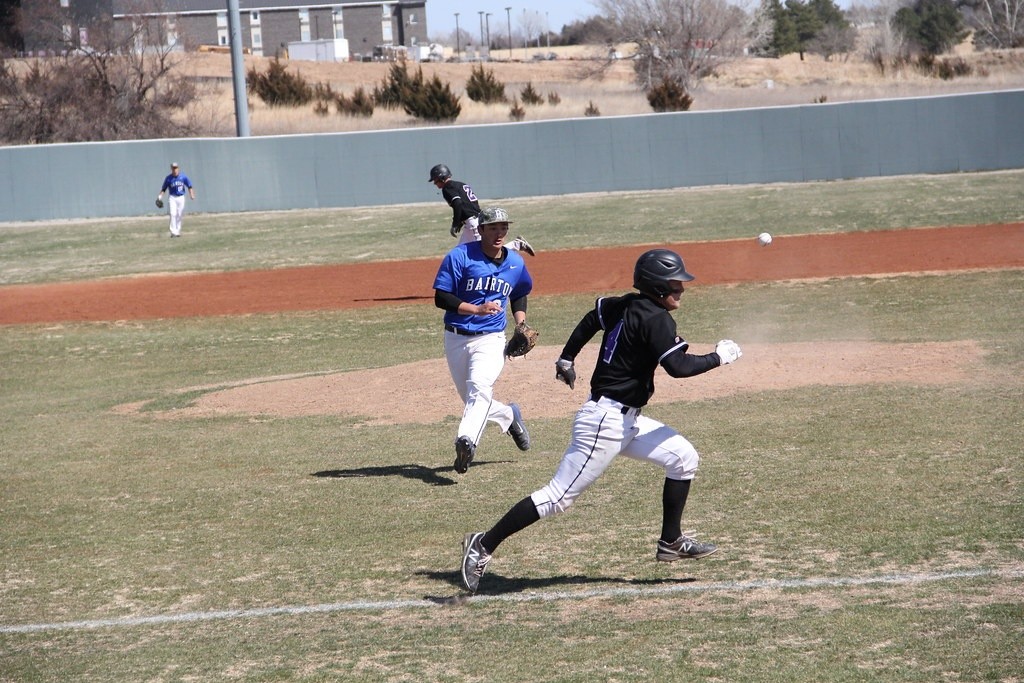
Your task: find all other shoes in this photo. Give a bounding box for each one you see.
[171,234,180,238]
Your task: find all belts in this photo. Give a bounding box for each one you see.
[443,325,492,336]
[463,216,476,225]
[591,393,641,418]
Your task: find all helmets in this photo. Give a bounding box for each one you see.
[633,249,695,301]
[428,163,451,184]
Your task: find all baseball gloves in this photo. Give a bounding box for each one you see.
[156,199,164,208]
[504,322,539,357]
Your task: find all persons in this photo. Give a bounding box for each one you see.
[460,248,743,594]
[157,162,194,238]
[427,164,535,257]
[432,209,532,474]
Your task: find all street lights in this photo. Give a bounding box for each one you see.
[546,12,550,47]
[455,12,460,55]
[504,7,513,56]
[485,13,491,56]
[479,12,484,46]
[536,11,540,47]
[523,8,528,48]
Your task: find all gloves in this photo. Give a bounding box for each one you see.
[715,340,743,366]
[556,359,577,390]
[451,223,464,238]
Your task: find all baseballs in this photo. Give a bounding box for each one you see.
[758,233,772,247]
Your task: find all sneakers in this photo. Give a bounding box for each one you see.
[657,535,719,561]
[460,532,493,593]
[454,436,475,473]
[515,236,535,256]
[506,404,530,451]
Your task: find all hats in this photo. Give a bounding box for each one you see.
[478,205,514,227]
[171,163,178,169]
